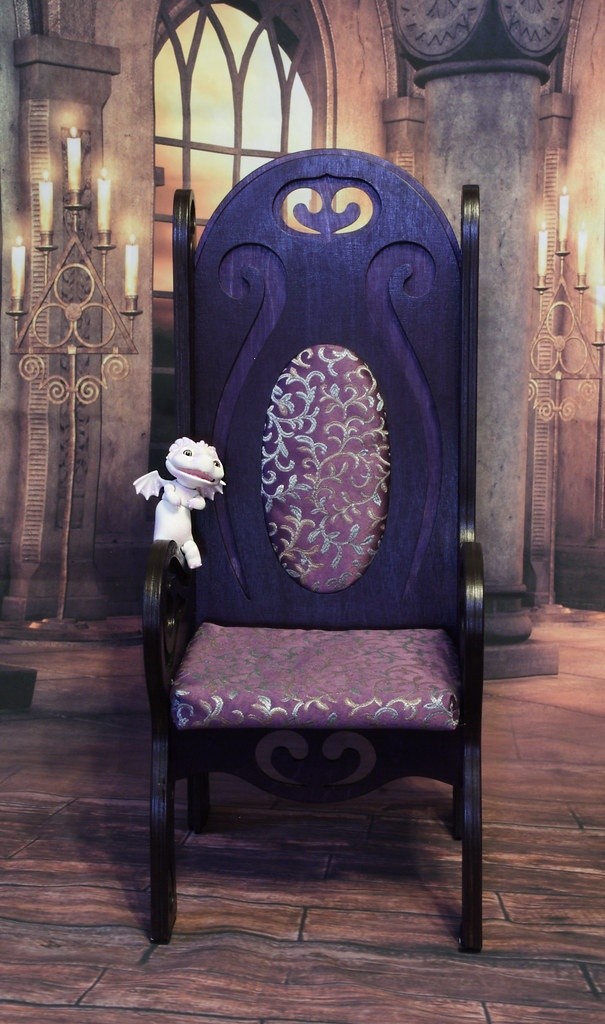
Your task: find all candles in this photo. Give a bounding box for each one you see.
[67,128,82,191]
[559,186,568,242]
[124,236,139,296]
[98,168,112,232]
[537,221,547,276]
[578,228,588,275]
[38,172,53,233]
[595,278,605,332]
[11,237,25,299]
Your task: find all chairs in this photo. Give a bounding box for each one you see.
[142,148,485,953]
